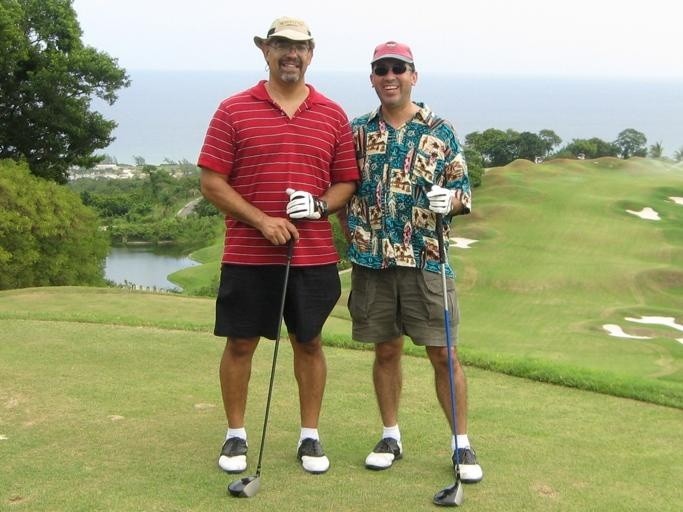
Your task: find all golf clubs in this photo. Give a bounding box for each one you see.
[432,215,463,507]
[227,219,297,496]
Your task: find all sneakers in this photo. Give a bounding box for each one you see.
[218,437,249,473]
[365,437,402,471]
[452,448,483,482]
[297,438,329,474]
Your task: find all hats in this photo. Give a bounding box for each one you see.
[254,17,315,49]
[371,40,414,64]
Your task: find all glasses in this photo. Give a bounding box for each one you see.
[375,66,406,75]
[274,41,307,51]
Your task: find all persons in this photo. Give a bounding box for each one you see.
[197,17,361,475]
[336,41,483,484]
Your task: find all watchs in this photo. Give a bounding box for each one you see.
[315,198,327,218]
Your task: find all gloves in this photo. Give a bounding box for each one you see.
[286,188,328,219]
[426,185,453,215]
